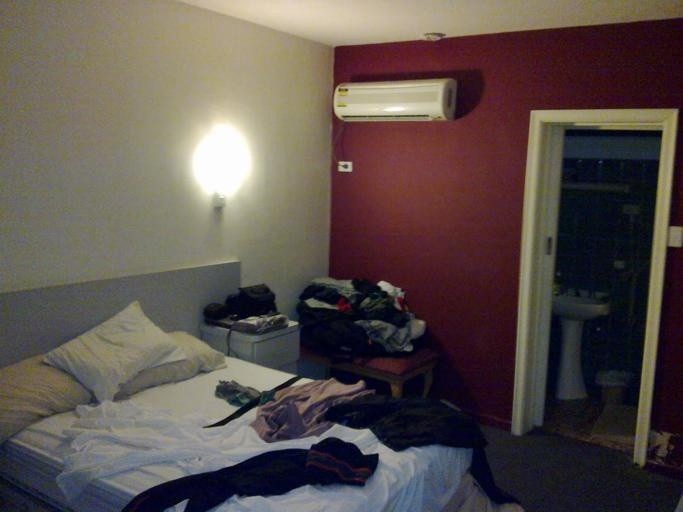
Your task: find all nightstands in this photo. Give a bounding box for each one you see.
[202,320,303,376]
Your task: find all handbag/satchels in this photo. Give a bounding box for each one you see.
[226,282,281,321]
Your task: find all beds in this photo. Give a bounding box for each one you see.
[2,336,491,511]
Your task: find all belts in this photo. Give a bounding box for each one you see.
[202,374,304,428]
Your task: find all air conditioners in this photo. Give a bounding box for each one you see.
[333,79,457,124]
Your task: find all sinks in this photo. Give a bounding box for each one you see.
[553,295,610,319]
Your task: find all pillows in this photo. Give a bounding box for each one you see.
[117,328,227,398]
[1,356,97,443]
[40,299,188,406]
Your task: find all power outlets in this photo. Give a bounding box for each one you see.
[338,161,353,172]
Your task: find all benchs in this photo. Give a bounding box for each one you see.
[303,336,440,399]
